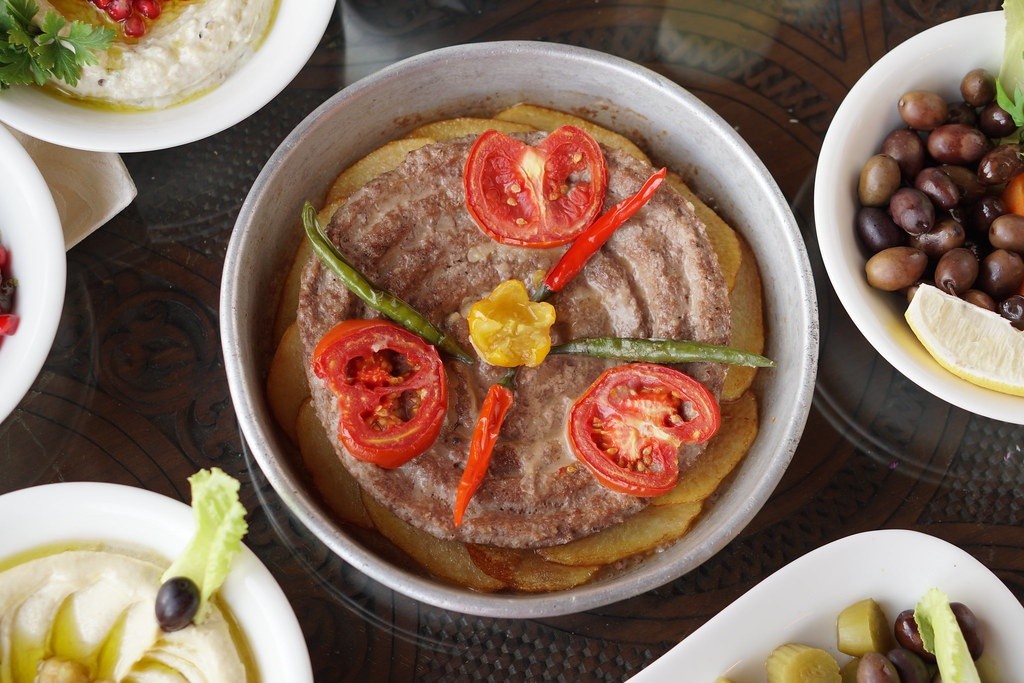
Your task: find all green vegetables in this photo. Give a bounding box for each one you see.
[994,0,1024,128]
[0,0,117,88]
[162,465,248,627]
[914,586,980,683]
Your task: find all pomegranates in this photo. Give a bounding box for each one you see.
[86,0,168,38]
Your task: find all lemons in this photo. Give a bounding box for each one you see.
[904,282,1024,398]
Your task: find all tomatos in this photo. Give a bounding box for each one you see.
[312,320,449,469]
[565,363,720,497]
[463,123,605,250]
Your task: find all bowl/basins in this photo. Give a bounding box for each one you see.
[815,10,1023,427]
[0,0,336,154]
[220,38,820,619]
[0,123,67,423]
[0,482,314,683]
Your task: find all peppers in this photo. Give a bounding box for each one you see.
[303,197,475,365]
[547,336,777,368]
[530,166,667,301]
[454,367,520,526]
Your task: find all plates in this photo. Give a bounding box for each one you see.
[622,527,1024,683]
